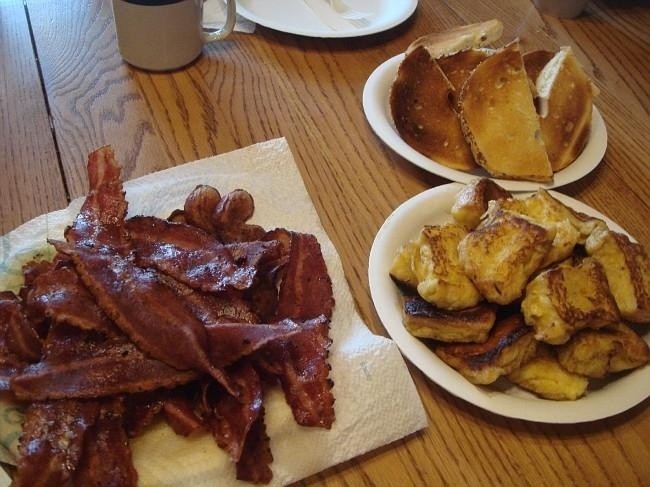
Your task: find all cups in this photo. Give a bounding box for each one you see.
[112,0,236,71]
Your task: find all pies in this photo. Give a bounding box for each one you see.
[390,175,650,401]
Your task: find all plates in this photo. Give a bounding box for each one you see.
[223,0,419,38]
[363,47,608,192]
[368,181,650,423]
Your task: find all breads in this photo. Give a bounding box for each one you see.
[386,19,600,183]
